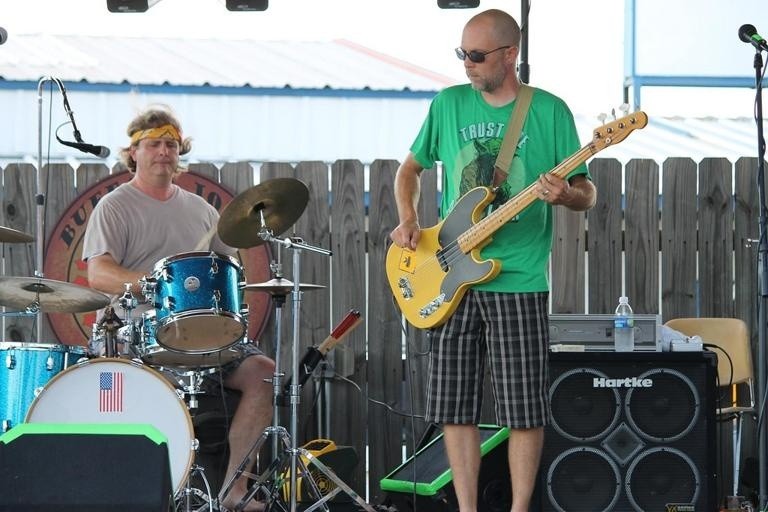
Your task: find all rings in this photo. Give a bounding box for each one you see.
[543,190,550,195]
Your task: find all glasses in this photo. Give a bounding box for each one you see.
[455,46,511,62]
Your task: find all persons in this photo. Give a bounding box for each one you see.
[391,9,598,512]
[82,111,276,510]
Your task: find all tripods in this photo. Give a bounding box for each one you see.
[240,258,374,511]
[216,295,329,511]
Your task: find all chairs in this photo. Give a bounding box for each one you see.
[663,319,754,498]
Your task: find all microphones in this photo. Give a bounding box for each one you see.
[737,24,766,50]
[57,136,111,161]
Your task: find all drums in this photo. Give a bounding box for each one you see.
[136,309,248,377]
[137,250,249,354]
[0,342,97,437]
[24,356,199,502]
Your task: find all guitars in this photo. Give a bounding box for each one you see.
[385,103,648,328]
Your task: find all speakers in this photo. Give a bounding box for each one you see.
[378,421,510,511]
[538,350,719,512]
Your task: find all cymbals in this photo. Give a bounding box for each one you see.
[218,178,309,249]
[0,275,111,313]
[0,225,37,243]
[245,279,325,294]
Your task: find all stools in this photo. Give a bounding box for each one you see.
[180,387,262,508]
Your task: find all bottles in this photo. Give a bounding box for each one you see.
[614,297,634,352]
